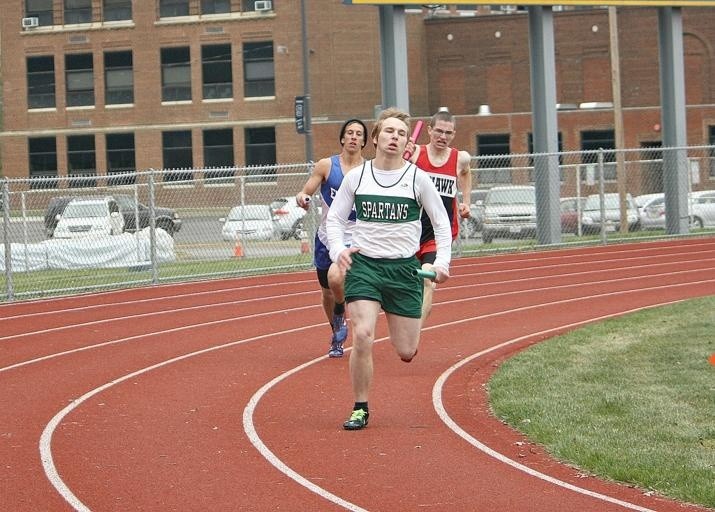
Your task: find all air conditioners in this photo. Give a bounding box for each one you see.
[21,16,38,30]
[254,0,273,13]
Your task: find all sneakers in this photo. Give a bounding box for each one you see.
[327,340,345,359]
[329,312,348,342]
[342,409,372,431]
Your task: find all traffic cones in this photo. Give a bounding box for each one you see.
[232,239,245,258]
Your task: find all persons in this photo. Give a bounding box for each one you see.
[294,119,369,358]
[325,108,453,430]
[401,110,472,362]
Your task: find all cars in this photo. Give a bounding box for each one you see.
[52,196,126,240]
[456,184,715,244]
[218,195,323,242]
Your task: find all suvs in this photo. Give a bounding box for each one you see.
[40,194,183,237]
